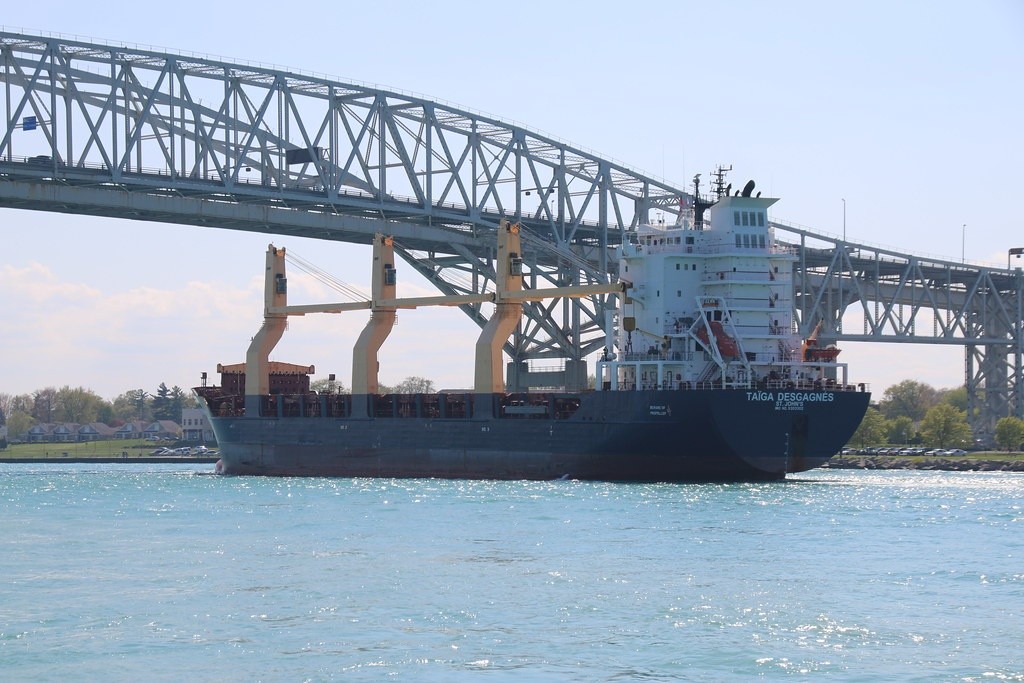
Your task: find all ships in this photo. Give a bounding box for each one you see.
[190,173,872,488]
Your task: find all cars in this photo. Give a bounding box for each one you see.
[194,445,207,452]
[856,446,916,456]
[174,436,180,441]
[149,446,191,457]
[915,447,934,456]
[925,447,947,457]
[842,447,858,456]
[146,436,160,441]
[202,450,218,456]
[164,437,170,441]
[191,452,205,457]
[943,448,967,457]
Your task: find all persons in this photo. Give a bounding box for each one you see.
[122,451,128,458]
[673,319,680,334]
[603,346,608,361]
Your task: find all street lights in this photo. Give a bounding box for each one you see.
[166,147,169,175]
[841,196,847,240]
[962,224,967,263]
